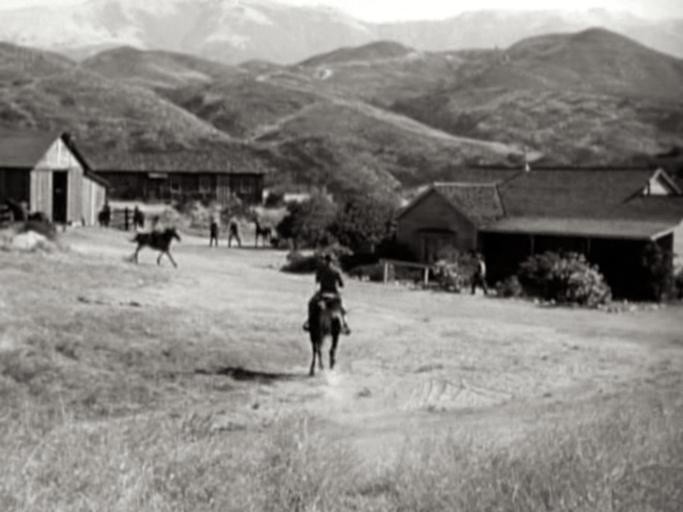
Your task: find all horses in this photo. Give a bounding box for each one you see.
[123,225,183,268]
[250,216,273,248]
[303,290,344,376]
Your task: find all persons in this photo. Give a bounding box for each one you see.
[303,253,350,335]
[209,215,273,248]
[471,253,487,295]
[97,199,165,248]
